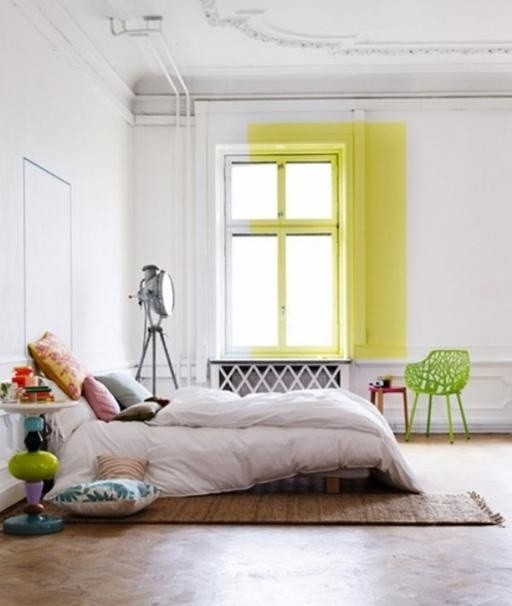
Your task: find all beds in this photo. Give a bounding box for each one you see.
[26,332,419,499]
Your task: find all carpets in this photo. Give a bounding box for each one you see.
[63,489,504,525]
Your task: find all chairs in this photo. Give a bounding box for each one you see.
[402,350,473,444]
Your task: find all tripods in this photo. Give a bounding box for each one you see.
[136,327,177,398]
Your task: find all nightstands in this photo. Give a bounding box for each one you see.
[0,392,81,535]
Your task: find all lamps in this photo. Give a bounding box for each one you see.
[135,264,177,392]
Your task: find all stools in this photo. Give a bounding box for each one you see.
[366,384,409,437]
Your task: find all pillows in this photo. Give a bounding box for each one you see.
[27,332,169,426]
[52,455,158,517]
